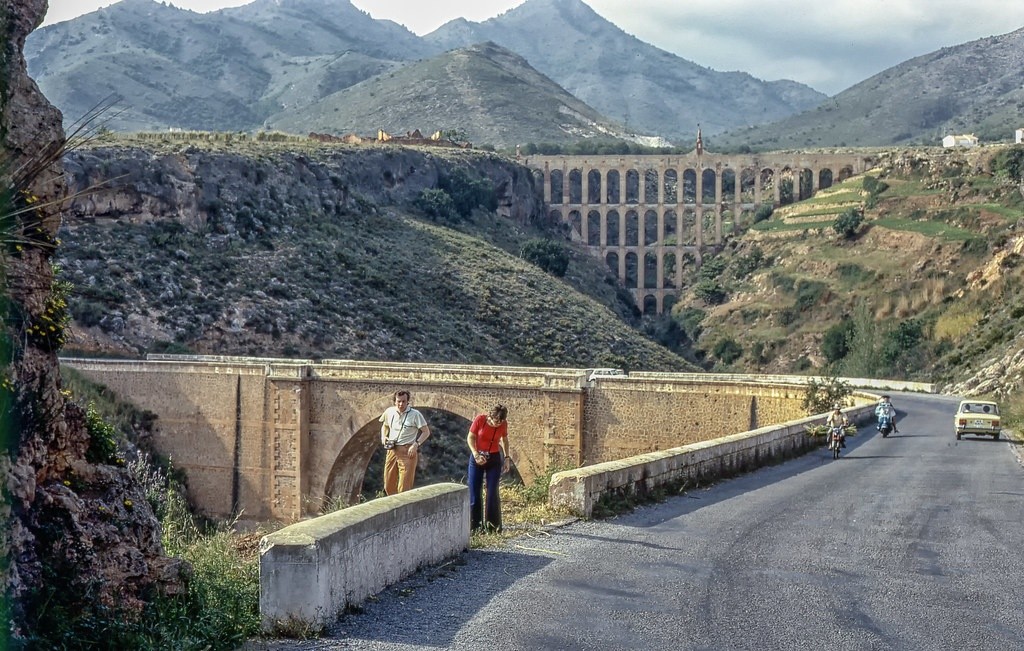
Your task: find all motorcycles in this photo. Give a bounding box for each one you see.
[828,425,845,459]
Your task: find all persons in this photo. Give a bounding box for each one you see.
[378,389,430,496]
[983,405,991,415]
[876,395,899,434]
[964,404,973,413]
[826,404,848,450]
[468,404,510,534]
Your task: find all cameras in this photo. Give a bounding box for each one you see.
[383,440,396,450]
[478,451,490,460]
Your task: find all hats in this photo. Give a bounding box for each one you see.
[833,404,841,410]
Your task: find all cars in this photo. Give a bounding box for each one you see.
[954,400,1002,440]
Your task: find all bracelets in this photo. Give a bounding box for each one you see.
[473,452,479,458]
[416,441,420,447]
[504,456,510,459]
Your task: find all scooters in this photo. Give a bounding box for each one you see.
[876,417,892,439]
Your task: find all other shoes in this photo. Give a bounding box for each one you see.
[877,426,881,430]
[895,430,899,433]
[828,447,832,450]
[842,445,846,448]
